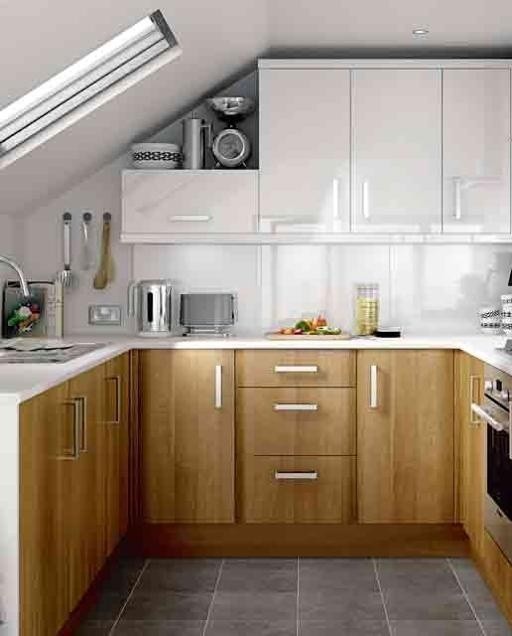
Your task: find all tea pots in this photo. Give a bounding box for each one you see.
[180,116,212,170]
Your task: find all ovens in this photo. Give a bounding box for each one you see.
[471,363,512,567]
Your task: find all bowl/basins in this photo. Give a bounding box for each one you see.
[131,143,179,169]
[479,294,512,334]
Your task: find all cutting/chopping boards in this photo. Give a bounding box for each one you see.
[267,333,351,340]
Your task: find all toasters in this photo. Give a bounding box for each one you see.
[179,293,236,337]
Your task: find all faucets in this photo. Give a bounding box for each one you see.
[0,252,31,300]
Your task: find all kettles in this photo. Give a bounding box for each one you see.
[126,279,171,338]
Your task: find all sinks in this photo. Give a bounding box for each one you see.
[0,363,44,393]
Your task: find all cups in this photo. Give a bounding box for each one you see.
[353,283,379,340]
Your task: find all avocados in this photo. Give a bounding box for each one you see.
[296,321,309,332]
[317,324,341,335]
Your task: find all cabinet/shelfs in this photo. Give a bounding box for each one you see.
[456,348,486,570]
[358,348,455,524]
[258,66,440,244]
[105,349,137,558]
[234,347,357,526]
[441,67,512,244]
[1,363,106,636]
[120,169,259,244]
[138,346,235,525]
